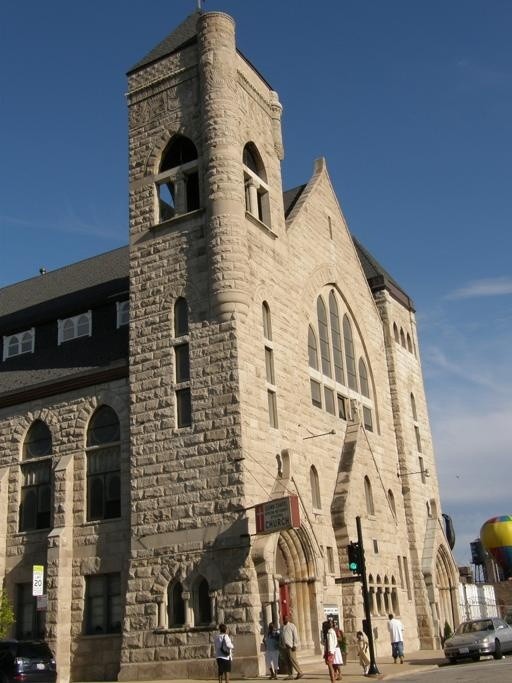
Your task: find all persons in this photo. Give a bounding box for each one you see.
[387,614,405,664]
[278,615,304,680]
[214,624,234,683]
[263,623,280,679]
[322,621,370,683]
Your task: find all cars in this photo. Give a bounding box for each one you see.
[443,616,512,664]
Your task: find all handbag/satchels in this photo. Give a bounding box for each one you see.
[220,634,231,656]
[324,654,335,665]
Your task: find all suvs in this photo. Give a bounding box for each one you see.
[0,638,59,682]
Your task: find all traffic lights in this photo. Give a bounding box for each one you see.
[346,540,368,576]
